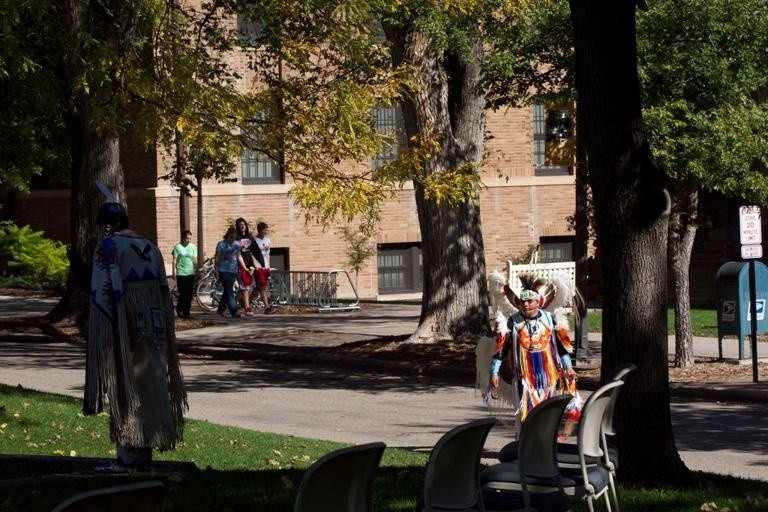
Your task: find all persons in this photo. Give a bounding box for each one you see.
[248,223,276,314]
[213,225,249,320]
[172,230,204,319]
[83,202,190,469]
[232,218,273,315]
[489,288,580,433]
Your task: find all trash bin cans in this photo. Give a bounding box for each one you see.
[717,261,767,364]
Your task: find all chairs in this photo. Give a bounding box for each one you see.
[471,393,575,511]
[288,442,386,511]
[502,361,639,511]
[416,416,500,512]
[46,476,166,510]
[557,380,626,511]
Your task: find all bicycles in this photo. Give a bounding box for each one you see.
[163,256,282,314]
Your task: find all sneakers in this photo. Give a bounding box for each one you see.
[217,310,227,318]
[264,305,276,315]
[177,311,193,318]
[232,311,241,318]
[244,307,255,316]
